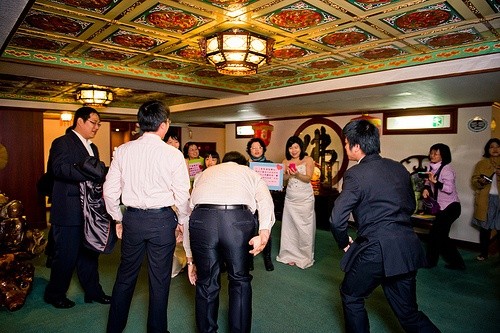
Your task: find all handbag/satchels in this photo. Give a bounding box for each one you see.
[423,195,441,214]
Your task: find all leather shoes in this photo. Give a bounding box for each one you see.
[84,293,111,304]
[44,293,76,309]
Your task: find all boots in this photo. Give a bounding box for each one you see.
[263,240,274,271]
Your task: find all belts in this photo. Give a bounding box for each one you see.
[196,204,248,210]
[124,206,172,213]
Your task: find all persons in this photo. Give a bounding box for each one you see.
[244,137,284,272]
[42,107,112,309]
[275,135,317,270]
[182,141,205,197]
[202,151,221,172]
[183,151,276,333]
[102,100,191,333]
[327,119,442,333]
[419,143,465,270]
[469,138,500,261]
[165,134,182,154]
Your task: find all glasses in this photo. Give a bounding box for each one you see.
[87,119,101,127]
[250,146,263,150]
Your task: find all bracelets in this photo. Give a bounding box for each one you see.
[186,261,194,265]
[116,221,122,224]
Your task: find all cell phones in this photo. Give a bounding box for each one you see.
[417,172,431,179]
[480,174,493,183]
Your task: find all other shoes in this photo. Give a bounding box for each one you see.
[477,256,485,260]
[444,263,465,271]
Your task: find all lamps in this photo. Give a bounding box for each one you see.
[204,26,276,76]
[72,86,117,108]
